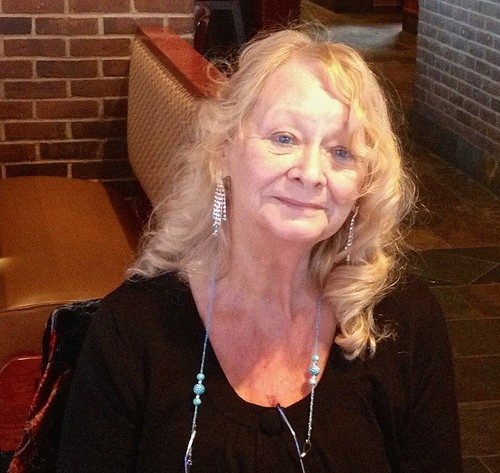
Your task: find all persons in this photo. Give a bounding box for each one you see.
[56,31,462,473]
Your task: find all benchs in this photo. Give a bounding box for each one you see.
[0,26,229,452]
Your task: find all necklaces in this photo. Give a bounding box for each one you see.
[182,237,325,473]
